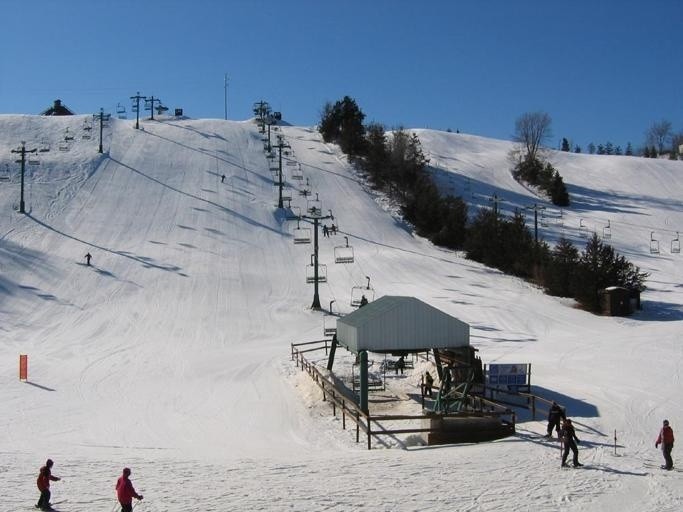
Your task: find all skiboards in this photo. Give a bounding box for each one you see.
[35,500,67,511]
[528,435,564,441]
[560,466,586,469]
[642,462,683,473]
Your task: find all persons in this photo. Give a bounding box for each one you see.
[84,252,93,266]
[394,357,406,375]
[358,295,368,309]
[330,224,336,236]
[221,173,225,183]
[425,371,435,396]
[543,400,567,439]
[323,225,329,238]
[303,189,307,197]
[309,206,315,215]
[655,419,675,471]
[115,467,144,512]
[558,418,584,468]
[37,458,62,512]
[285,150,289,156]
